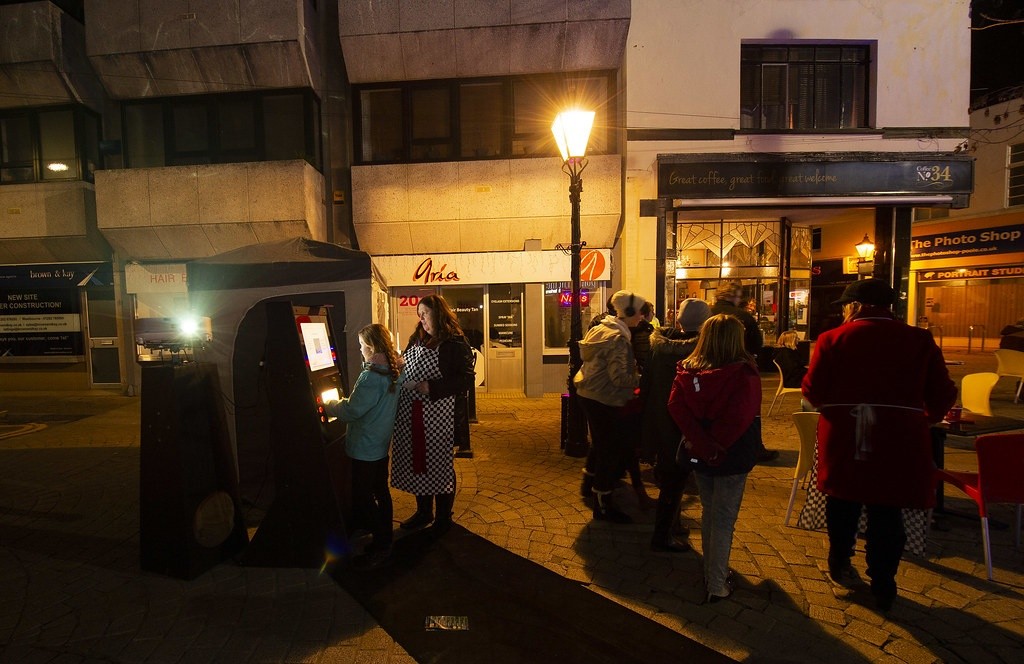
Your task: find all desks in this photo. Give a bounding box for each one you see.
[930,412,1024,531]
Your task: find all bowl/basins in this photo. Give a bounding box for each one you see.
[944,407,963,423]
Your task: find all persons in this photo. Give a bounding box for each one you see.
[666,314,764,603]
[322,324,402,553]
[390,295,474,539]
[802,278,959,611]
[1000,319,1024,402]
[774,330,808,388]
[572,281,768,554]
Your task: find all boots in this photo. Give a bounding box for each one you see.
[581,468,596,498]
[591,486,634,524]
[429,494,454,542]
[400,493,433,532]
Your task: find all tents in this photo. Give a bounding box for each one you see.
[187,237,378,557]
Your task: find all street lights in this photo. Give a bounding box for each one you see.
[550,103,595,456]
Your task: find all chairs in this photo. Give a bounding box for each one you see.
[961,372,999,417]
[783,412,821,526]
[928,428,1024,580]
[767,351,804,417]
[994,349,1024,403]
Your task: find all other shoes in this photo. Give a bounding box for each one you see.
[760,443,779,463]
[709,579,736,604]
[869,579,896,603]
[726,570,735,577]
[649,534,692,553]
[671,520,689,536]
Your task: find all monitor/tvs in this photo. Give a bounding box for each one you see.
[300,322,335,372]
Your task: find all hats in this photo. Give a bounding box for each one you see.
[830,279,897,305]
[641,302,654,317]
[610,291,644,317]
[677,298,710,332]
